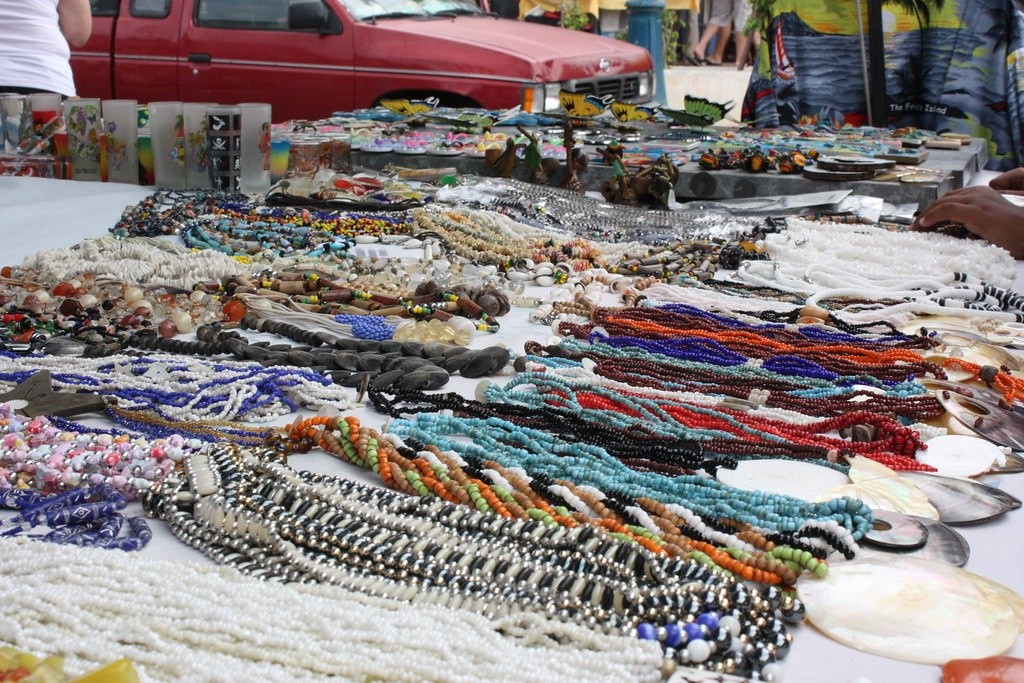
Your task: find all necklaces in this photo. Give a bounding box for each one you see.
[0,162,1021,679]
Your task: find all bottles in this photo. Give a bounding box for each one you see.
[0,93,30,154]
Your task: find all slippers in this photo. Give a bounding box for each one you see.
[684,57,708,65]
[706,60,720,66]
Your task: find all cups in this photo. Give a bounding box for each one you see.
[31,93,62,154]
[101,99,137,182]
[273,133,352,176]
[148,101,226,188]
[63,98,101,181]
[207,107,243,194]
[238,102,271,196]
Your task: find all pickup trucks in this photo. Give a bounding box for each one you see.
[65,0,665,123]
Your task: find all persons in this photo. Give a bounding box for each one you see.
[0,0,94,103]
[685,0,760,68]
[909,168,1024,261]
